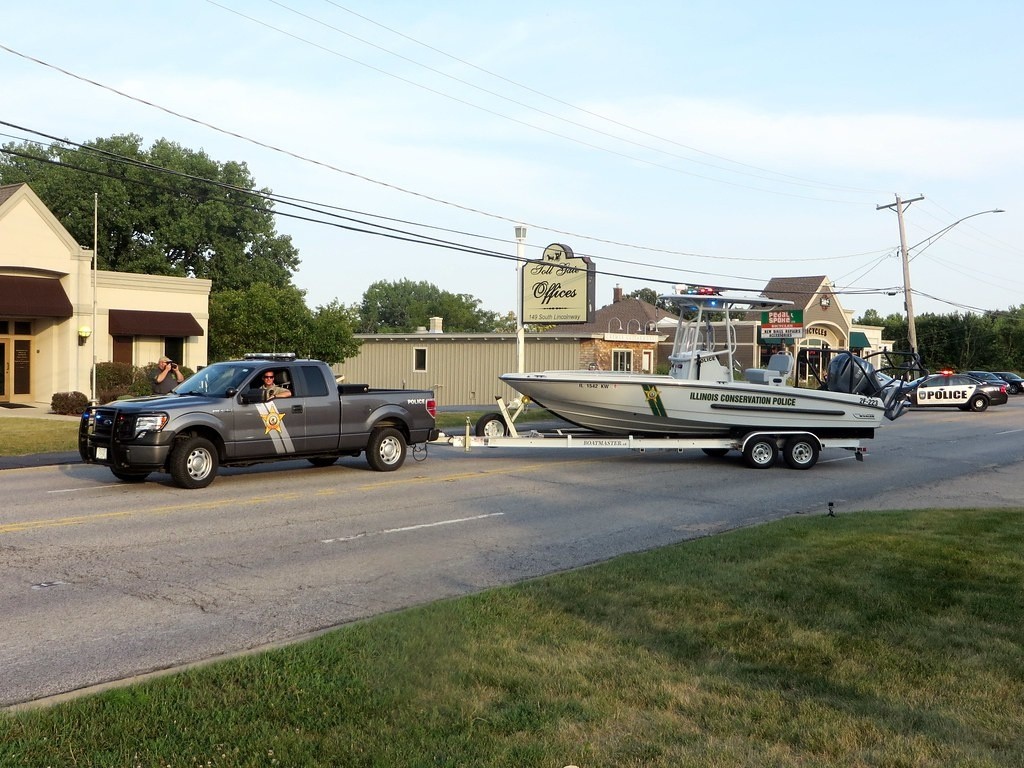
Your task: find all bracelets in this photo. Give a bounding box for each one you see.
[273,394,276,398]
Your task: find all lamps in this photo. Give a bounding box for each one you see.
[608,317,624,333]
[825,280,836,288]
[78,326,92,346]
[626,319,642,333]
[644,320,660,335]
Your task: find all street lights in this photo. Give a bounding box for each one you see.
[901,209,1006,380]
[511,220,529,409]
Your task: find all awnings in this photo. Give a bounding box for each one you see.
[757,325,794,346]
[109,310,204,337]
[850,332,871,351]
[0,275,73,317]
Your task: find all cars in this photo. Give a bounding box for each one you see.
[987,371,1024,395]
[958,370,1012,395]
[875,371,907,404]
[895,370,1009,412]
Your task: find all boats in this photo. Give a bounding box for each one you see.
[497,285,931,439]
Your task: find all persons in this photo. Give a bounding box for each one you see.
[254,369,292,403]
[151,356,184,396]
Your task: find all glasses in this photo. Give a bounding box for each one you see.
[264,376,274,378]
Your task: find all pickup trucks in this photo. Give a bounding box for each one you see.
[79,352,440,490]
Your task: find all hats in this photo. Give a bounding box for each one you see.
[159,357,172,363]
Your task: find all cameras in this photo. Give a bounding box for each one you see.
[167,362,177,370]
